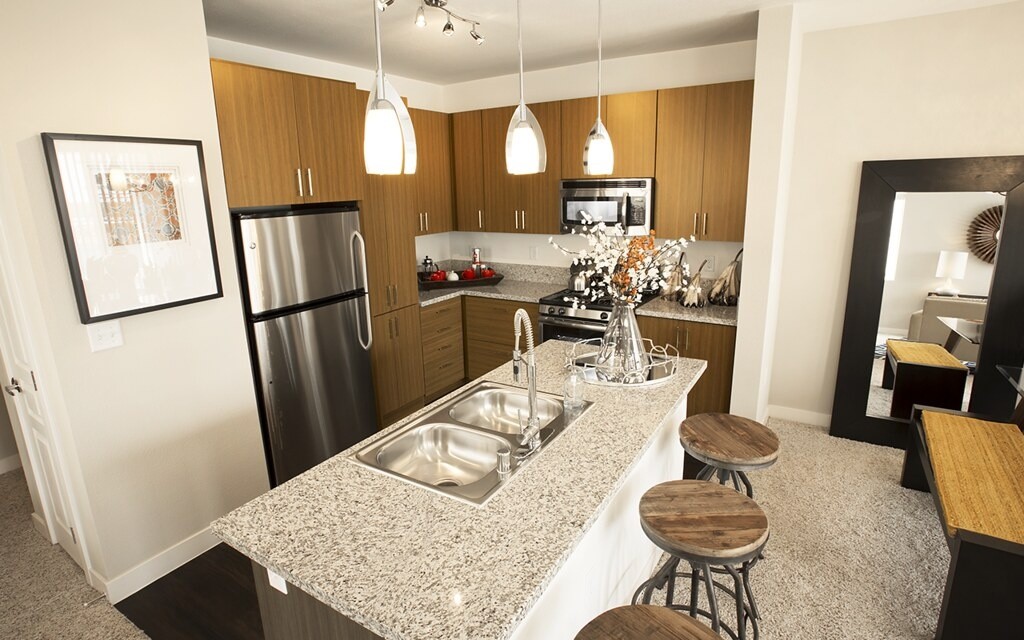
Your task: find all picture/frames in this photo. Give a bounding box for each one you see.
[38,129,226,325]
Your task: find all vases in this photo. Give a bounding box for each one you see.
[592,290,653,385]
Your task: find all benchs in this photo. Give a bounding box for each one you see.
[896,402,1024,640]
[881,336,972,422]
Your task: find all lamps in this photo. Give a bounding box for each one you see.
[359,0,421,178]
[503,0,548,177]
[375,0,486,46]
[934,248,969,295]
[581,0,615,177]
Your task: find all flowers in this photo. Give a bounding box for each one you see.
[545,211,703,374]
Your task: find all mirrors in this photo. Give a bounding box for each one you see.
[863,182,1012,428]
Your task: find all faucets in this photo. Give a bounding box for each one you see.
[509,306,541,450]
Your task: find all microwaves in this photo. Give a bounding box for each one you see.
[559,178,654,236]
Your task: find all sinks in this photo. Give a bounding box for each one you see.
[441,379,594,448]
[347,417,521,509]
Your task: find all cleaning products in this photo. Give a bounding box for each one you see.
[563,364,586,410]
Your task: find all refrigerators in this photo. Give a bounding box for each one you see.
[228,201,379,491]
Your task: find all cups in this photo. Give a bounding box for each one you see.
[430,270,459,281]
[563,371,585,410]
[461,266,494,280]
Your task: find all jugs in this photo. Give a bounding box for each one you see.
[422,255,439,272]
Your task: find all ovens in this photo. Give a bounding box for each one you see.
[538,315,608,346]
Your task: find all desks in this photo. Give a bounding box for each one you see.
[909,293,991,365]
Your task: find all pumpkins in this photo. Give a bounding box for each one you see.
[659,248,743,309]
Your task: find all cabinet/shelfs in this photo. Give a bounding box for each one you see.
[209,57,759,432]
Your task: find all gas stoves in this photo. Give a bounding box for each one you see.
[538,261,661,323]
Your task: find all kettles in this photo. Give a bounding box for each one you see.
[568,258,598,292]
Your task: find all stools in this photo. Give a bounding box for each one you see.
[677,410,785,503]
[572,478,773,640]
